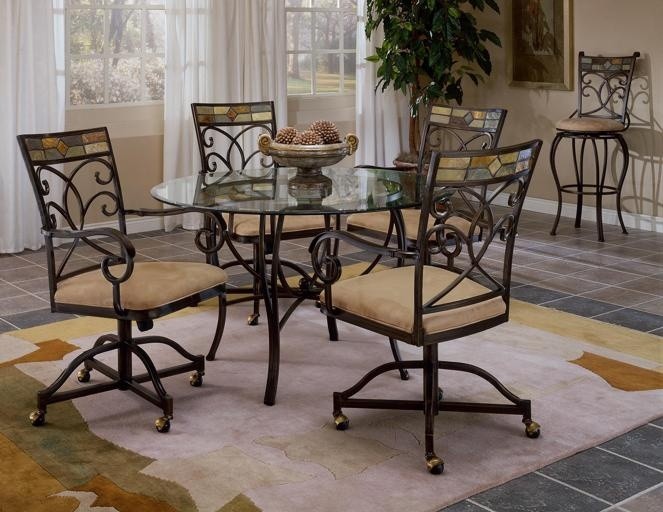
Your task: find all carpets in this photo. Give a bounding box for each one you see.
[0,262,662,512]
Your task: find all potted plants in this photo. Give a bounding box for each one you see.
[363,0,505,207]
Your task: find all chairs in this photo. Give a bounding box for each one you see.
[549,49,641,243]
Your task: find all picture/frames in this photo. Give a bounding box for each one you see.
[507,0,574,91]
